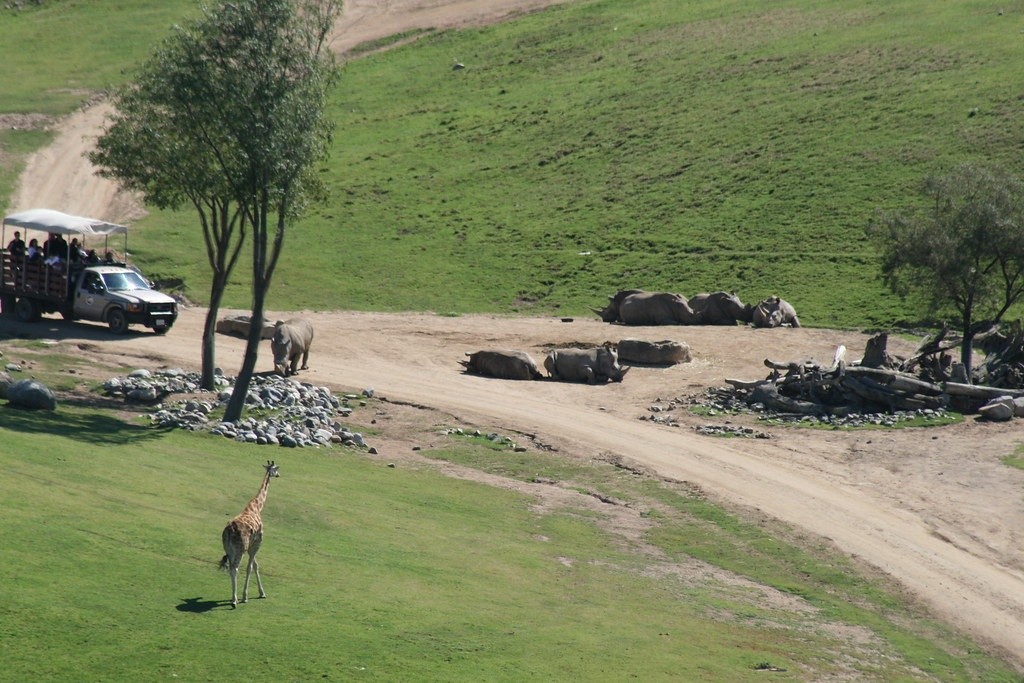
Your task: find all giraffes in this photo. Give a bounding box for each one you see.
[219,460,280,608]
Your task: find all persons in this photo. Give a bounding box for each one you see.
[28,231,116,276]
[6,230,25,276]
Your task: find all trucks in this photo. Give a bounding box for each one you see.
[0,209,179,335]
